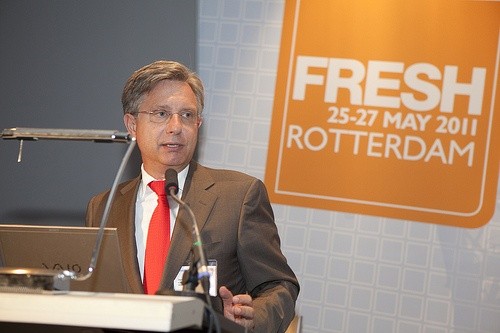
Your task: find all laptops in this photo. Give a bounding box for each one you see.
[0,225,131,294]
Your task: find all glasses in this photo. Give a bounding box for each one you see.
[126,109,202,127]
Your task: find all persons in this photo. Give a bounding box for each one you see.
[84,60,301,333]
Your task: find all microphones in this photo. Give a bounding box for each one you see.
[164,169,210,295]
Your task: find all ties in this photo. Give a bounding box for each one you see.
[142,180,172,295]
